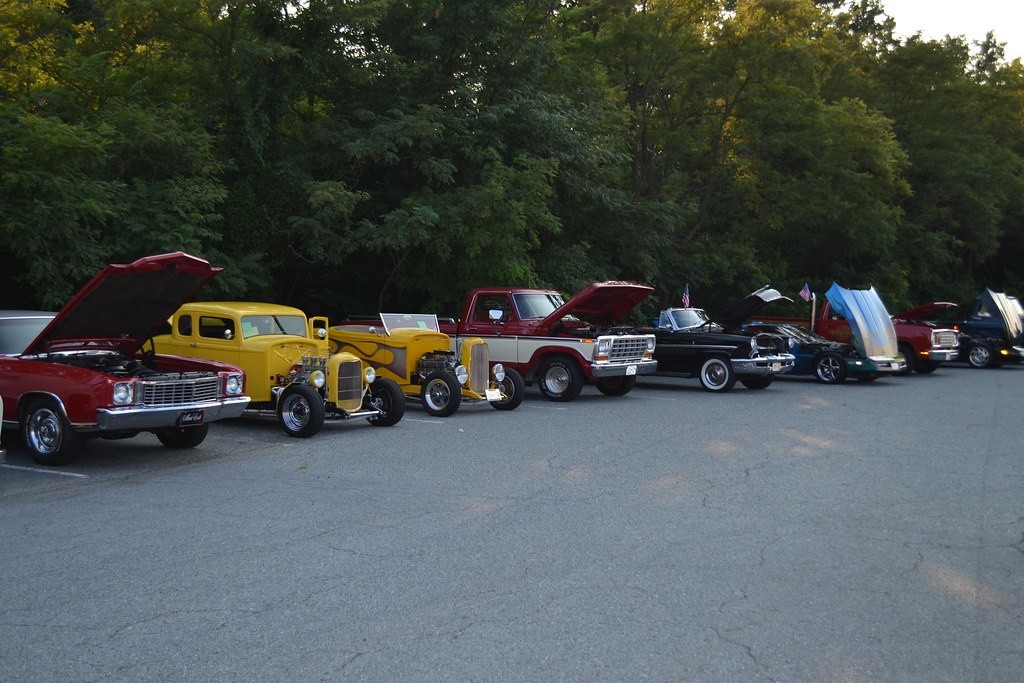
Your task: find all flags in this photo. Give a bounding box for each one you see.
[799,284,810,302]
[682,285,690,308]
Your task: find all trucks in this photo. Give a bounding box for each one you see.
[338,280,658,403]
[746,296,960,376]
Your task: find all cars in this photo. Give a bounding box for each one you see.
[739,282,906,385]
[327,313,525,418]
[135,301,405,437]
[0,252,250,464]
[931,287,1024,369]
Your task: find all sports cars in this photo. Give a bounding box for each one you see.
[630,285,796,393]
